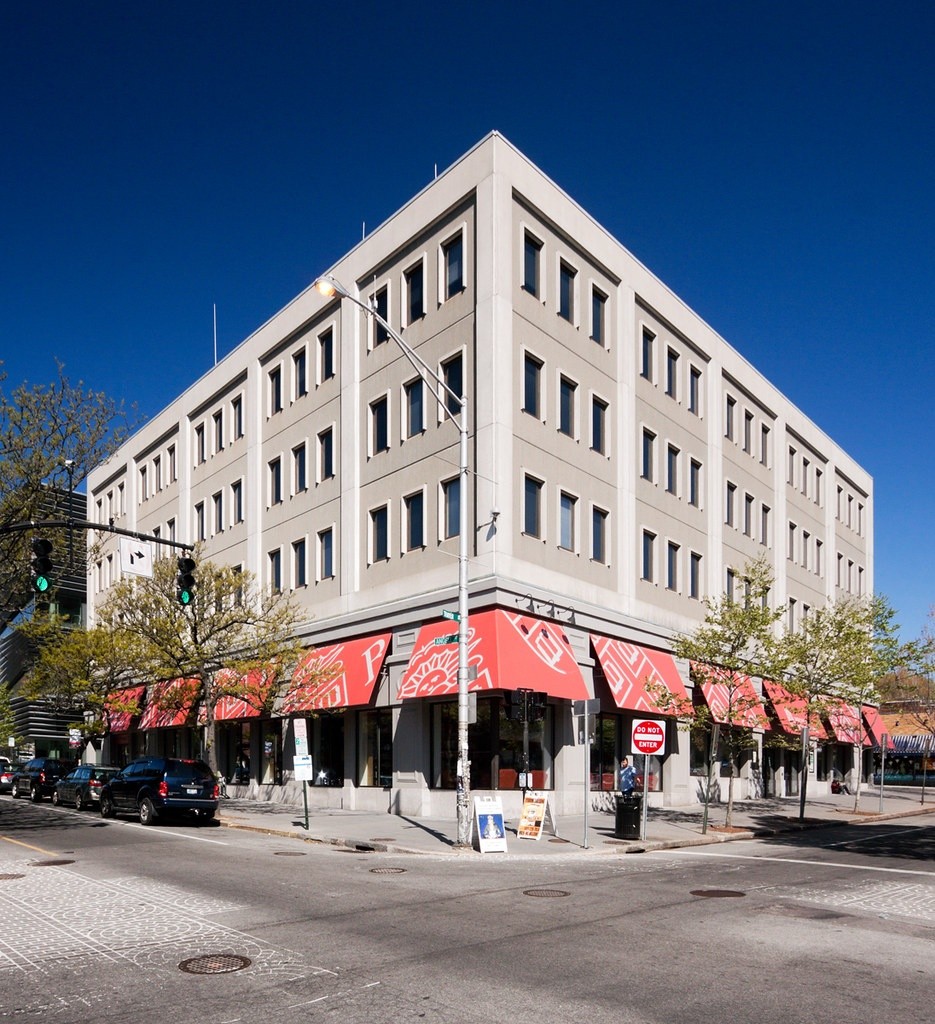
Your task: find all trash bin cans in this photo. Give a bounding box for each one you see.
[614,794,644,840]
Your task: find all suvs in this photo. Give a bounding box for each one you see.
[98,756,220,826]
[10,757,75,804]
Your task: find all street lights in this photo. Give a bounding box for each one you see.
[316,275,473,848]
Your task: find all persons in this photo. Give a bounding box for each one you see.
[618,758,636,796]
[832,779,851,795]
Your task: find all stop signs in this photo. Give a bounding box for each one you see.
[630,719,666,755]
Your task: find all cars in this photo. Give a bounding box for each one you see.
[51,763,121,812]
[0,755,26,794]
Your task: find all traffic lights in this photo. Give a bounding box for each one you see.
[528,691,548,722]
[32,538,53,594]
[177,557,196,606]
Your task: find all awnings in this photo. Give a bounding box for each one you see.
[818,694,872,746]
[873,734,935,753]
[99,683,146,732]
[689,660,772,730]
[763,680,829,739]
[282,631,393,712]
[201,655,278,721]
[397,608,590,700]
[861,706,895,748]
[589,633,695,715]
[137,674,201,729]
